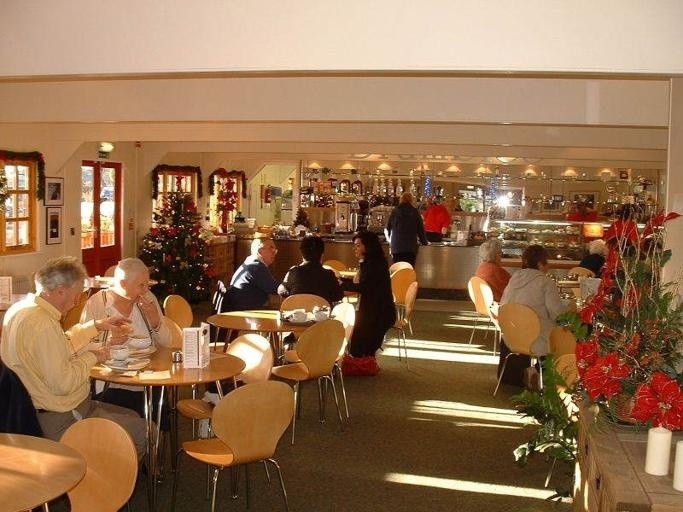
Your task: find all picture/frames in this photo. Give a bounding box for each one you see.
[44,177,64,206]
[46,207,62,245]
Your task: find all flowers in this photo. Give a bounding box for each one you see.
[556,210,683,432]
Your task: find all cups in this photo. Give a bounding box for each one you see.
[292,311,306,320]
[110,344,129,360]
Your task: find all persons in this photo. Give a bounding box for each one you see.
[421,201,449,243]
[277,236,344,307]
[0,256,158,466]
[79,258,182,418]
[476,240,512,302]
[384,192,428,269]
[607,205,639,246]
[500,245,577,356]
[578,239,606,278]
[339,231,397,357]
[230,237,279,311]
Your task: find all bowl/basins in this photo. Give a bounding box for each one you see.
[129,334,152,349]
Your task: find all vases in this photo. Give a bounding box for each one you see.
[604,394,639,425]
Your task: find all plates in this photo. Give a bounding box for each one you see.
[289,320,309,326]
[106,358,151,371]
[129,347,157,358]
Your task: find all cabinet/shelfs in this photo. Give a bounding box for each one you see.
[499,175,657,221]
[300,168,423,240]
[491,220,647,265]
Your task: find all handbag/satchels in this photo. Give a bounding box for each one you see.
[340,354,379,376]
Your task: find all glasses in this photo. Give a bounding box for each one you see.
[270,247,278,253]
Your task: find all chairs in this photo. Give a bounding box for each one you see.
[270,319,345,446]
[170,380,296,512]
[175,334,274,444]
[389,261,414,274]
[326,302,356,418]
[279,294,331,340]
[323,260,346,271]
[163,294,193,330]
[56,417,139,512]
[493,303,546,401]
[390,268,417,318]
[467,276,499,357]
[392,281,418,372]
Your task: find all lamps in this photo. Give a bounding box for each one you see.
[98,141,115,160]
